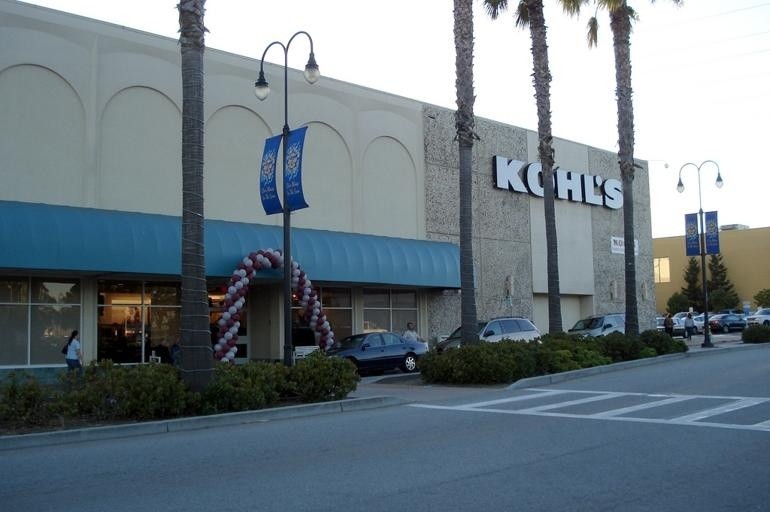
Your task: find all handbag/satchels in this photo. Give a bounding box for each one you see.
[61,344,68,354]
[685,332,688,337]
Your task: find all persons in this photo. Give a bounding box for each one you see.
[666,313,673,338]
[403,323,427,343]
[684,313,694,341]
[65,330,83,387]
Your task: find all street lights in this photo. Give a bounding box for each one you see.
[676,153,726,347]
[254,29,318,369]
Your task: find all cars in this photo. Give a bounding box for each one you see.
[700,311,716,319]
[320,331,429,376]
[363,321,388,334]
[709,313,747,335]
[656,316,689,338]
[719,308,749,316]
[683,315,705,334]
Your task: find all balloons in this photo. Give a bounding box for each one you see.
[214,248,335,365]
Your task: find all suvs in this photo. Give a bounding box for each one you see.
[431,317,542,352]
[673,311,699,319]
[570,313,627,337]
[743,307,770,330]
